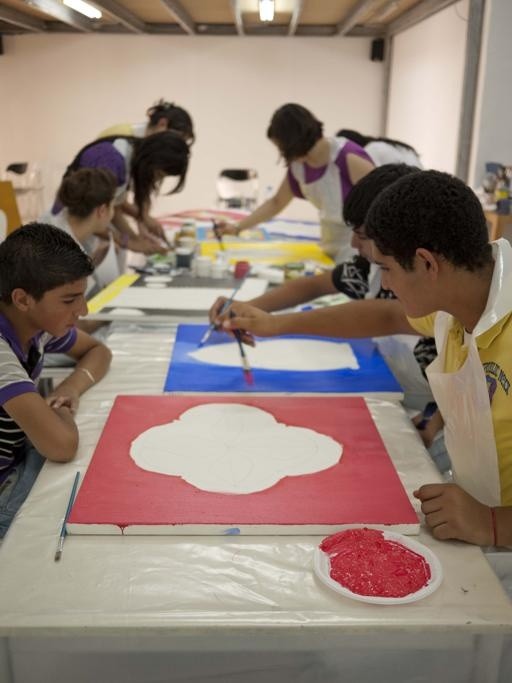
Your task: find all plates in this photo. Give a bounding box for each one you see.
[312,530,440,605]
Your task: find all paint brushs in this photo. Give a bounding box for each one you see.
[211,218,227,252]
[160,233,176,253]
[198,287,237,348]
[128,265,154,276]
[54,472,80,561]
[229,310,253,385]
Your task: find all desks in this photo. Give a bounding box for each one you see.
[1,285,512,683]
[480,195,512,242]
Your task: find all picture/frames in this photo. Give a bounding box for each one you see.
[156,209,248,224]
[269,219,321,240]
[78,274,269,323]
[162,323,405,402]
[65,396,421,535]
[197,227,271,241]
[202,243,335,268]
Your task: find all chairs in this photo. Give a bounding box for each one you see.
[220,169,257,208]
[40,353,75,396]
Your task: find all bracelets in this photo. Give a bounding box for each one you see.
[490,506,500,550]
[76,365,98,385]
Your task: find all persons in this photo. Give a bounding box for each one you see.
[213,102,379,272]
[52,131,189,245]
[206,170,469,450]
[0,224,111,478]
[220,169,512,553]
[20,171,121,303]
[96,106,196,149]
[338,127,423,176]
